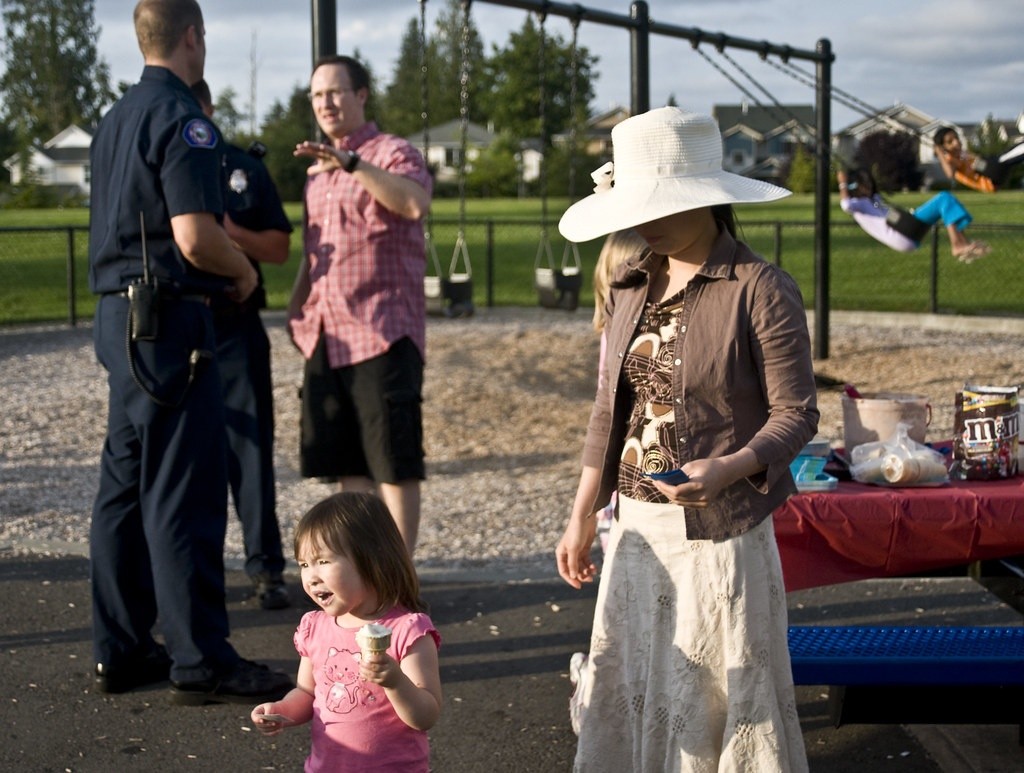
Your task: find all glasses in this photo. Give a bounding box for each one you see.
[306,88,356,102]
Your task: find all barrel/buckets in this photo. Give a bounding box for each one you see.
[839,392,932,463]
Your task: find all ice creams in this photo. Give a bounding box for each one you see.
[355,622,392,662]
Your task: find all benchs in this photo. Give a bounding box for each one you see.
[787,625,1023,724]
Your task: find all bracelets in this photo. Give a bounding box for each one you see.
[342,149,359,173]
[839,183,848,190]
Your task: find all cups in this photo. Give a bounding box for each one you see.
[359,630,392,665]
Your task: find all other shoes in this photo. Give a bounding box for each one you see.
[253,569,290,610]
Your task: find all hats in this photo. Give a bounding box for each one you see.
[559,105,795,244]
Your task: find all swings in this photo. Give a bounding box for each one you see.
[755,39,1011,188]
[689,26,933,247]
[532,0,587,314]
[420,0,476,317]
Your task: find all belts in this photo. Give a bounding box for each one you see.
[107,290,210,306]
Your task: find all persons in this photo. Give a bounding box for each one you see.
[836,168,993,264]
[86,0,296,708]
[285,55,434,561]
[933,126,1024,186]
[555,105,821,773]
[251,490,443,773]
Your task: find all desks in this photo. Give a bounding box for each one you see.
[777,437,1023,591]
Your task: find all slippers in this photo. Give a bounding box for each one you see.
[958,240,977,261]
[966,245,989,264]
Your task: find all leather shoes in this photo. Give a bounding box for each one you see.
[95,635,170,692]
[170,658,290,707]
